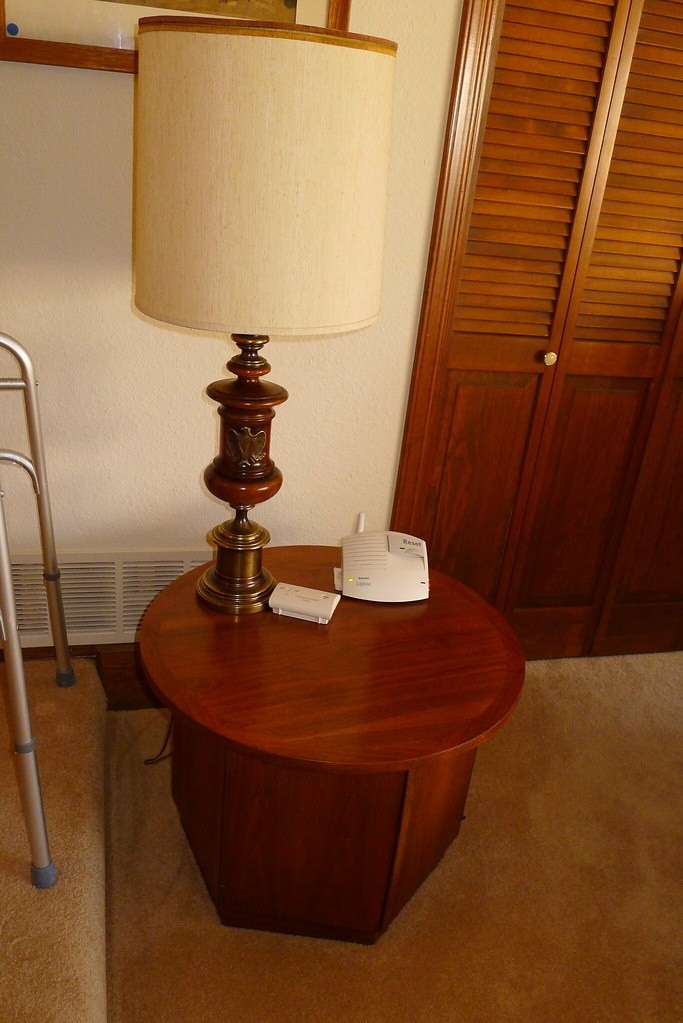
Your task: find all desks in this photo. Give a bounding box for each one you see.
[136,544,525,943]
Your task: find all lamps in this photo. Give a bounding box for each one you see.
[131,14,399,613]
